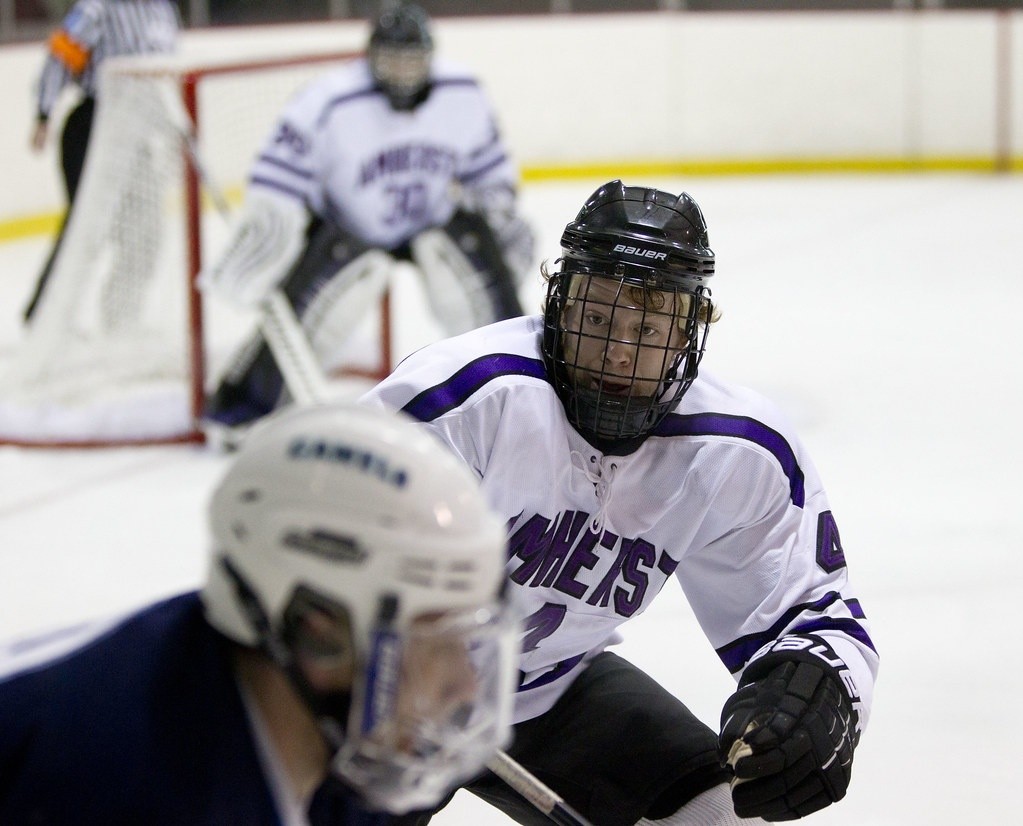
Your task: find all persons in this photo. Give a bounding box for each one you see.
[0,403,519,826]
[359,177,878,826]
[194,3,539,449]
[22,0,179,325]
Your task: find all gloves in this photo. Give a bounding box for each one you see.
[715,635,863,822]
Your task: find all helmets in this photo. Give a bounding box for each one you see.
[539,178,715,443]
[367,14,433,103]
[201,400,521,815]
